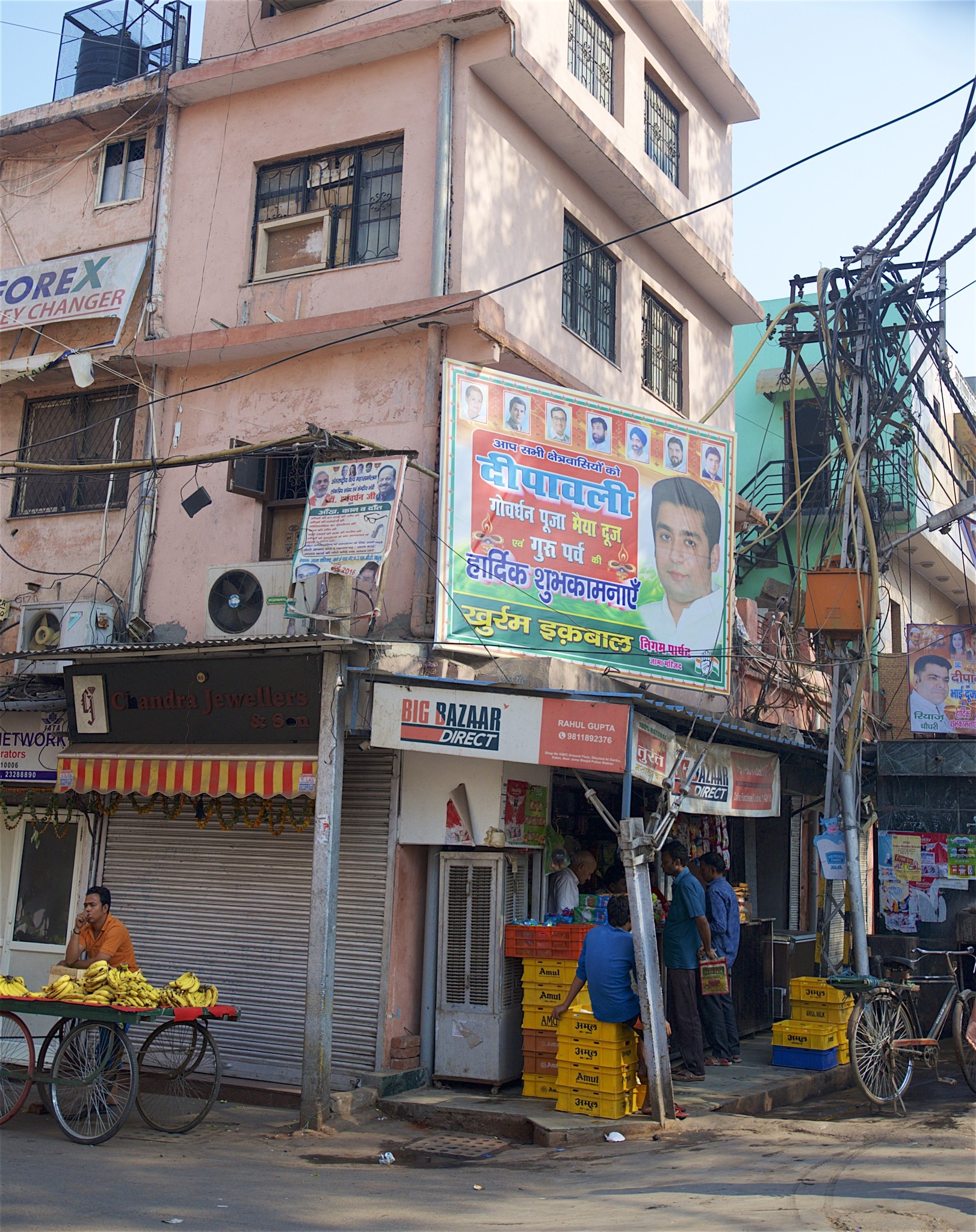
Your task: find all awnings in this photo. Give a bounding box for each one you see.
[53,743,318,800]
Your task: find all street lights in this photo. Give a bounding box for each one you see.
[868,496,976,737]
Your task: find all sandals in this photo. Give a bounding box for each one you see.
[732,1055,742,1063]
[704,1056,732,1066]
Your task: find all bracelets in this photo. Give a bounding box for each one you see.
[73,929,81,934]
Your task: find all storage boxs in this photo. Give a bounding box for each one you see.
[771,976,853,1071]
[502,924,646,1118]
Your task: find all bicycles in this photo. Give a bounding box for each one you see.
[825,947,976,1117]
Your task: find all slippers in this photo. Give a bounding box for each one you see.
[672,1070,705,1081]
[672,1065,685,1072]
[643,1108,689,1117]
[674,1103,686,1111]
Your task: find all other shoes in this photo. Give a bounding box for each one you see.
[83,1101,119,1112]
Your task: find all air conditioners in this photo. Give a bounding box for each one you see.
[12,602,115,675]
[204,559,317,642]
[960,479,976,501]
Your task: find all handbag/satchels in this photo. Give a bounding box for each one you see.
[682,949,699,996]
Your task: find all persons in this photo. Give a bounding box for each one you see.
[639,477,723,658]
[309,471,330,506]
[506,397,526,432]
[295,563,320,583]
[660,841,742,1080]
[588,417,609,453]
[547,851,597,914]
[366,463,372,473]
[341,465,348,477]
[462,386,485,422]
[551,895,680,1115]
[628,427,648,462]
[908,628,976,734]
[349,464,356,477]
[547,407,570,443]
[57,886,140,1113]
[376,465,396,502]
[702,447,722,481]
[356,561,379,584]
[665,437,685,472]
[594,865,669,906]
[357,464,364,474]
